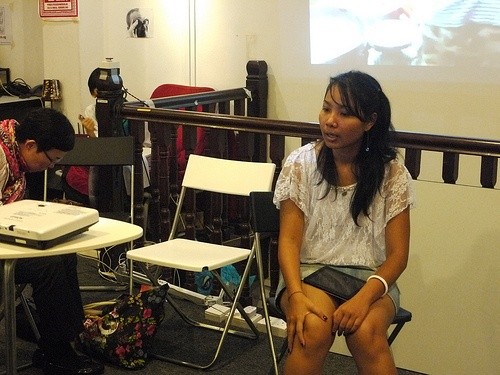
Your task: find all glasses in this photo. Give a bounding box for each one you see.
[43,150,57,165]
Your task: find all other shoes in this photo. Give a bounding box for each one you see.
[32,349,100,375]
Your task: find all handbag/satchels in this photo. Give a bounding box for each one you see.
[73,284,170,371]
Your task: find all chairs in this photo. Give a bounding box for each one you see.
[250,191,413,375]
[126,153,280,375]
[43,137,135,297]
[67,84,216,196]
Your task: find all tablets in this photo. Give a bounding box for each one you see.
[303,266,366,302]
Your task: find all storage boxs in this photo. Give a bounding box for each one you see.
[0,200,99,250]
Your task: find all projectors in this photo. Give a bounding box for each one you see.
[0,199,99,250]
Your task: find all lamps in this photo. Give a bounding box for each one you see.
[40,79,62,108]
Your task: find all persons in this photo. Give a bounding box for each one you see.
[79,66,130,207]
[0,107,106,375]
[274,70,416,375]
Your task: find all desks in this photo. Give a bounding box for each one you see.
[0,96,43,122]
[0,217,144,375]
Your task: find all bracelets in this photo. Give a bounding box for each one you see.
[288,290,304,301]
[366,274,389,298]
